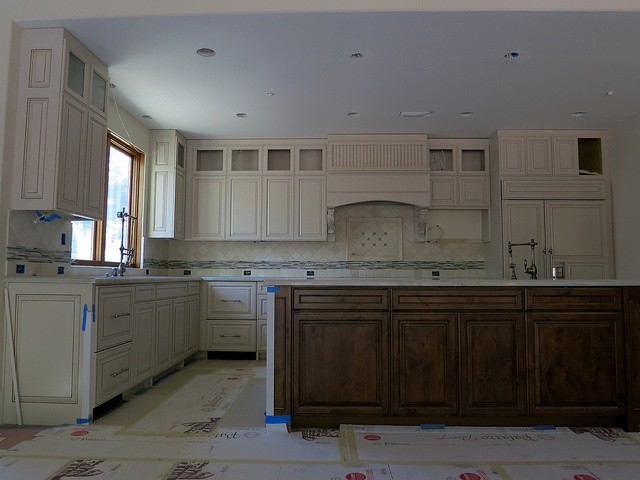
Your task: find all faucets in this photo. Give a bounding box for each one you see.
[105,207,138,278]
[505,239,540,280]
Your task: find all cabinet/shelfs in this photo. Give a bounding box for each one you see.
[485,178,615,277]
[147,135,187,239]
[1,342,136,426]
[524,287,625,425]
[188,146,262,238]
[258,283,270,351]
[429,138,488,212]
[0,283,135,353]
[390,286,525,428]
[293,288,391,427]
[261,144,325,241]
[554,135,603,176]
[135,283,156,388]
[205,321,257,352]
[7,39,111,222]
[205,282,257,318]
[624,285,640,432]
[274,285,292,415]
[489,136,554,177]
[156,283,188,376]
[188,282,200,354]
